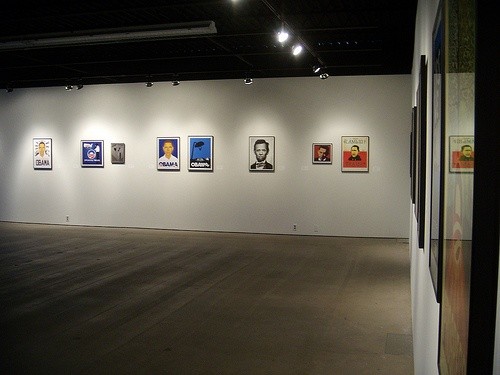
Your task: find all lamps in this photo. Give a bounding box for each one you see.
[145,82,153,87]
[64,85,83,92]
[243,78,252,85]
[312,57,330,79]
[172,81,180,86]
[0,21,217,51]
[274,15,302,56]
[6,88,14,93]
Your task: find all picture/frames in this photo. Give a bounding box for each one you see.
[249,136,275,171]
[33,138,52,169]
[188,135,213,171]
[341,135,369,172]
[449,135,474,172]
[156,137,180,170]
[80,139,103,167]
[405,0,500,375]
[312,142,333,164]
